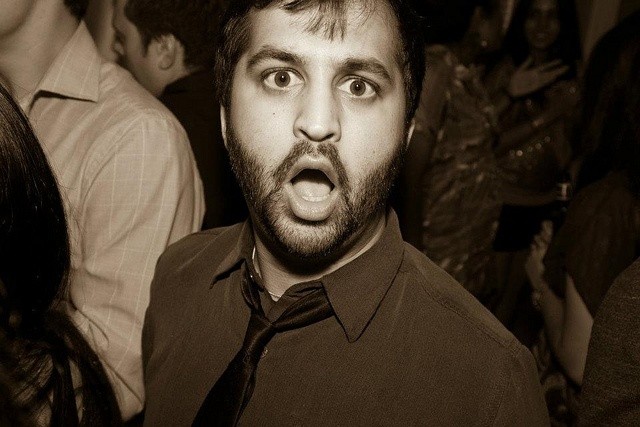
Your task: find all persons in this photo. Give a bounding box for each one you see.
[140,0,551,426]
[0,0,206,427]
[406,0,570,298]
[0,75,125,427]
[488,0,585,325]
[524,6,639,427]
[111,0,246,232]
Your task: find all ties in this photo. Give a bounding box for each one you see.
[195,270,335,426]
